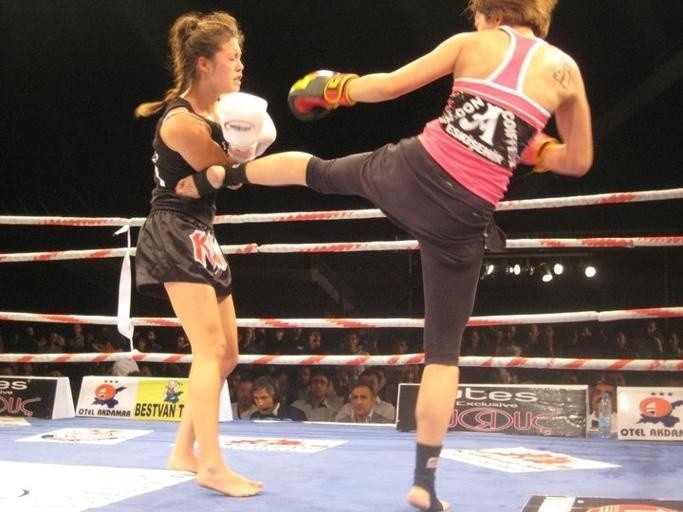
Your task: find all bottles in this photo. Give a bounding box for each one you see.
[598,393,612,439]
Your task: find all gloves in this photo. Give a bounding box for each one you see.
[288,70,358,123]
[217,93,276,164]
[519,129,556,179]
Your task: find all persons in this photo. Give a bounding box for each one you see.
[0,259,683,434]
[136,12,277,498]
[172,1,595,512]
